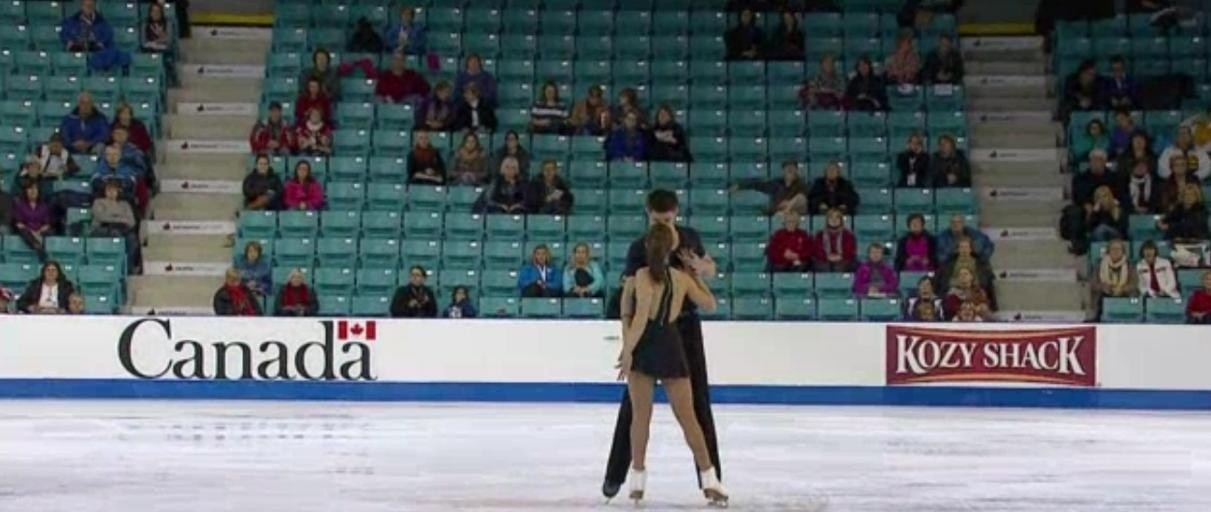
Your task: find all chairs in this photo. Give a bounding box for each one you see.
[711,226,730,321]
[646,0,730,189]
[613,0,646,283]
[679,189,731,225]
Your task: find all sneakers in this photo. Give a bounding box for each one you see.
[629,470,646,498]
[699,466,729,502]
[603,479,621,497]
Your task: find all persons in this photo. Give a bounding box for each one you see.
[275,268,320,316]
[0,287,14,314]
[933,214,995,267]
[392,266,438,317]
[16,262,75,314]
[444,286,476,318]
[722,8,772,61]
[1136,240,1180,298]
[928,136,971,185]
[59,0,112,51]
[291,106,335,155]
[563,241,606,296]
[249,101,289,154]
[10,152,65,233]
[140,3,179,87]
[598,189,723,497]
[570,84,612,132]
[59,91,113,154]
[924,35,965,85]
[375,53,430,101]
[1185,272,1211,325]
[895,133,933,189]
[277,158,324,210]
[520,244,562,296]
[35,133,75,181]
[213,268,264,316]
[815,207,857,271]
[905,275,944,320]
[381,4,428,53]
[236,240,274,295]
[727,158,811,217]
[484,155,527,214]
[603,109,650,161]
[851,243,901,298]
[1060,107,1210,254]
[800,53,846,107]
[406,129,447,184]
[945,269,992,320]
[610,88,650,127]
[1059,55,1140,128]
[764,210,815,270]
[295,78,332,120]
[650,104,694,161]
[111,99,151,160]
[808,162,860,214]
[494,128,535,175]
[64,286,84,314]
[89,143,141,217]
[448,132,493,184]
[1091,238,1139,325]
[886,29,922,84]
[531,80,571,134]
[241,153,284,209]
[454,79,500,130]
[614,224,730,500]
[903,5,940,40]
[300,49,341,102]
[892,213,937,270]
[772,11,806,61]
[842,55,891,111]
[416,81,460,130]
[452,54,498,107]
[526,159,575,214]
[96,123,148,179]
[87,178,141,275]
[8,178,54,264]
